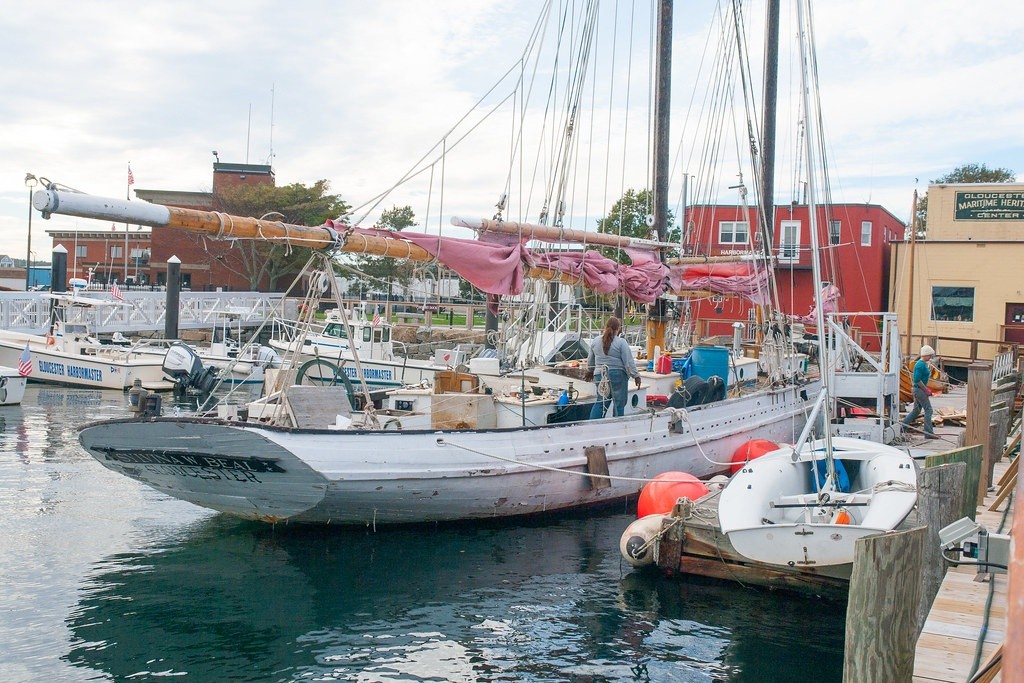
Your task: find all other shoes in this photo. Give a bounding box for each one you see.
[925,433,940,439]
[901,426,915,432]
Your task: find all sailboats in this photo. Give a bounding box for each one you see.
[1,2,947,607]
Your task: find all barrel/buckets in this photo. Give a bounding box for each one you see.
[692,346,729,399]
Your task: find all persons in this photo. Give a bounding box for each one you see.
[583,316,641,420]
[900,345,941,440]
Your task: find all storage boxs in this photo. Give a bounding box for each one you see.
[248,386,347,427]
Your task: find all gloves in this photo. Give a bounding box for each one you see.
[635,376,641,389]
[583,372,594,382]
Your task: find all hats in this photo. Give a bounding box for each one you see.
[921,345,935,356]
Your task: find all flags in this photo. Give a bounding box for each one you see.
[373,304,380,327]
[110,281,125,301]
[128,167,135,187]
[18,342,32,376]
